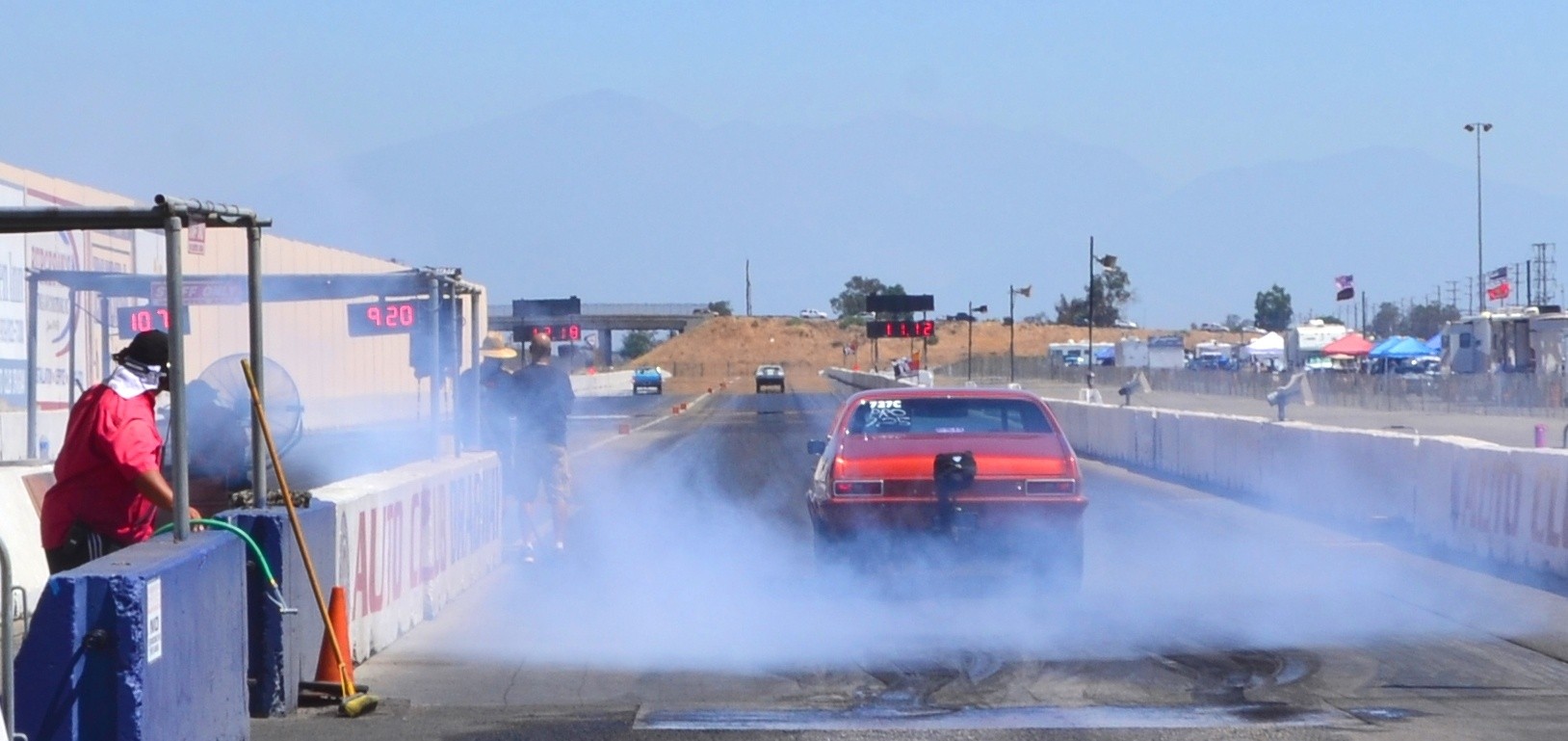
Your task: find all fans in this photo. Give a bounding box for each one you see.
[197,353,303,471]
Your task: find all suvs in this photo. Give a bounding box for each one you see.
[632,366,662,394]
[754,363,787,393]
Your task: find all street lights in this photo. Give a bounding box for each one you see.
[1087,235,1118,392]
[968,301,987,380]
[1008,284,1031,384]
[1465,122,1493,313]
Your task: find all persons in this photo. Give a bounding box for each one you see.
[877,405,910,432]
[40,328,206,576]
[509,333,576,564]
[449,329,518,452]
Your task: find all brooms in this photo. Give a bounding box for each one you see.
[239,358,375,717]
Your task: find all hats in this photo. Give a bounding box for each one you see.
[112,328,170,392]
[480,330,517,359]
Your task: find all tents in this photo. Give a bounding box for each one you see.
[1324,330,1441,374]
[1245,331,1284,368]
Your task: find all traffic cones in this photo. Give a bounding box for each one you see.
[296,586,371,694]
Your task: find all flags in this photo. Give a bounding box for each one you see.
[1335,275,1353,289]
[1488,284,1511,301]
[1337,288,1354,300]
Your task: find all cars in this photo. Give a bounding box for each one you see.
[805,385,1089,576]
